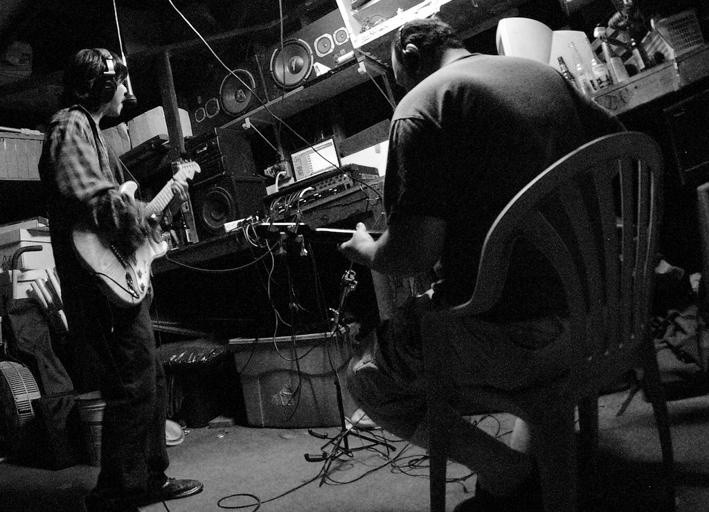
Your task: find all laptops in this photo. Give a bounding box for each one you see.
[278,136,341,190]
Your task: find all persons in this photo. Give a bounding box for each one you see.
[336,17,626,511]
[37,47,204,508]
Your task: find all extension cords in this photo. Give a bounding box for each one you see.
[224,216,260,234]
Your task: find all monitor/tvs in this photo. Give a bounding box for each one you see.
[496,17,597,87]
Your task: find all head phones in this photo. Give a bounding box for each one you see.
[395,24,421,78]
[93,47,117,103]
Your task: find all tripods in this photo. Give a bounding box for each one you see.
[319,377,397,487]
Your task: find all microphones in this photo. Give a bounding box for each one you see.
[122,53,137,105]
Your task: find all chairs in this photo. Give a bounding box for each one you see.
[422,132,675,511]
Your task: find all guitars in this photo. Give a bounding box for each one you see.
[238,215,388,256]
[71,158,202,307]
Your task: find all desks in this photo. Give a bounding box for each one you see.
[151,187,392,322]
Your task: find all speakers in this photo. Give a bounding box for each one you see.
[255,6,355,100]
[187,59,268,136]
[184,127,268,242]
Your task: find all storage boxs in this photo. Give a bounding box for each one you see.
[1,228,51,272]
[101,107,193,159]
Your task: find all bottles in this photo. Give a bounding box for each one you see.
[557,26,648,98]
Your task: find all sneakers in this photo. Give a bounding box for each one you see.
[454,452,535,512]
[151,479,203,499]
[539,444,576,512]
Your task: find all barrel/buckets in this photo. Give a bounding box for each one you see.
[76,401,107,468]
